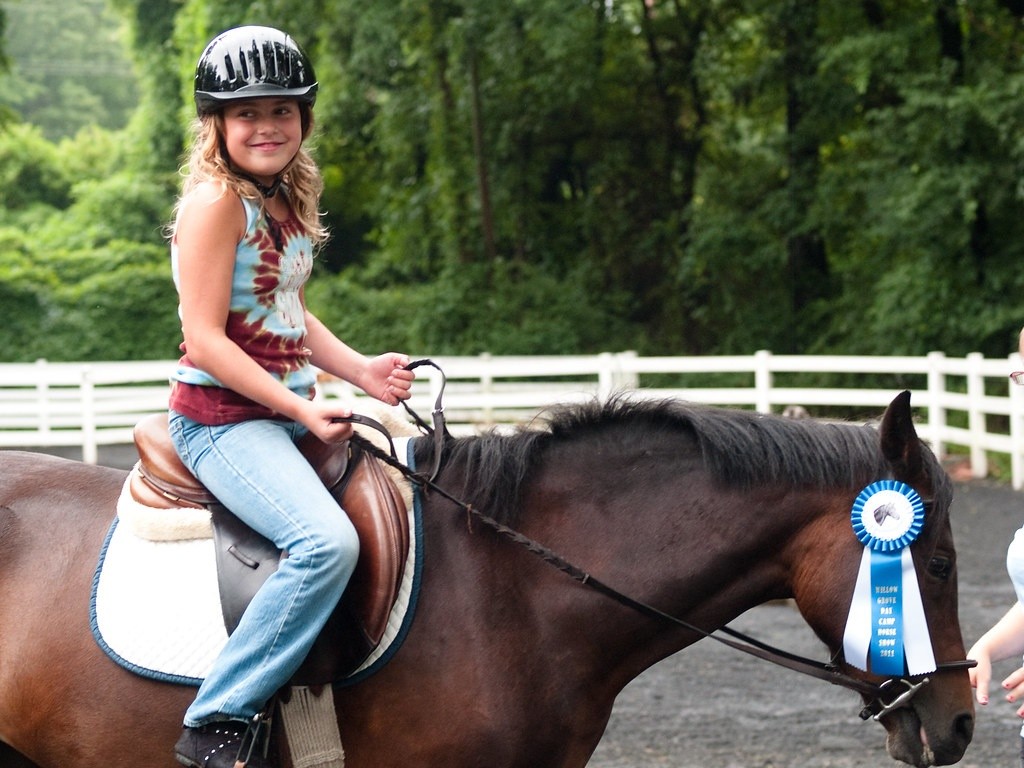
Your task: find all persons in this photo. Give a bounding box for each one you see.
[168,25,415,768]
[965,327,1024,768]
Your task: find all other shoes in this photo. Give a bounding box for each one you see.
[175,721,267,768]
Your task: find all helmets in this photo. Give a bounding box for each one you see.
[194,26,319,120]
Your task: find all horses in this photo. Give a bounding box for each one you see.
[0,388,976,768]
